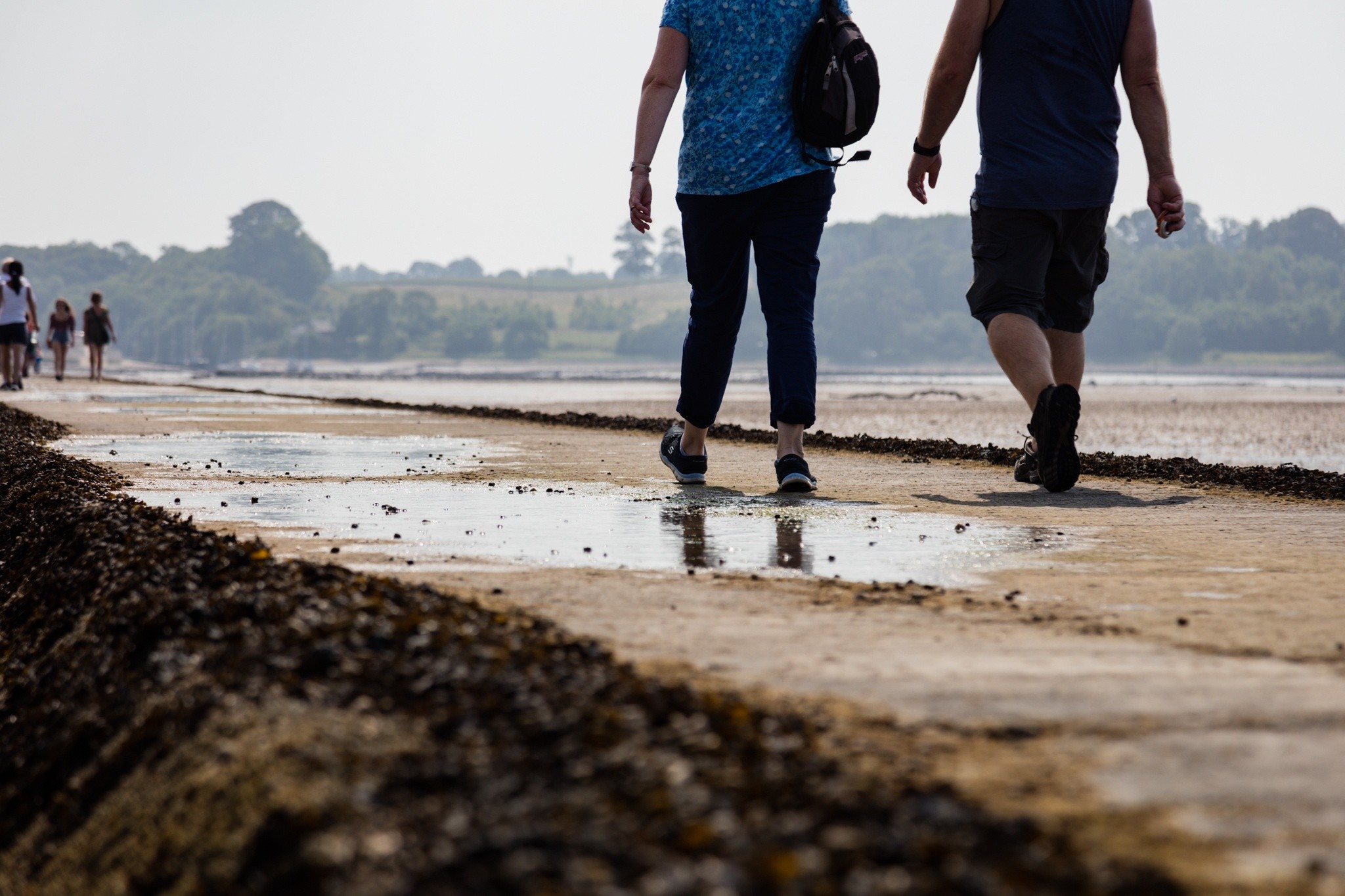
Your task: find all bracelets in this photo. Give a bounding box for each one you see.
[913,136,941,156]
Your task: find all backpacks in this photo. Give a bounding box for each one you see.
[792,1,881,165]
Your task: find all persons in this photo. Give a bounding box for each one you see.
[83,291,117,380]
[908,1,1185,493]
[627,0,852,492]
[0,256,43,391]
[46,298,76,382]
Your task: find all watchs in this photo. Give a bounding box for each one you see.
[629,162,651,172]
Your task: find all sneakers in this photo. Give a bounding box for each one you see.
[1027,384,1082,492]
[1014,454,1040,484]
[775,454,818,491]
[659,427,708,482]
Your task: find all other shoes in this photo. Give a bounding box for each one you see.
[12,382,23,392]
[1,383,11,390]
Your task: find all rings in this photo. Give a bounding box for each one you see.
[630,206,635,211]
